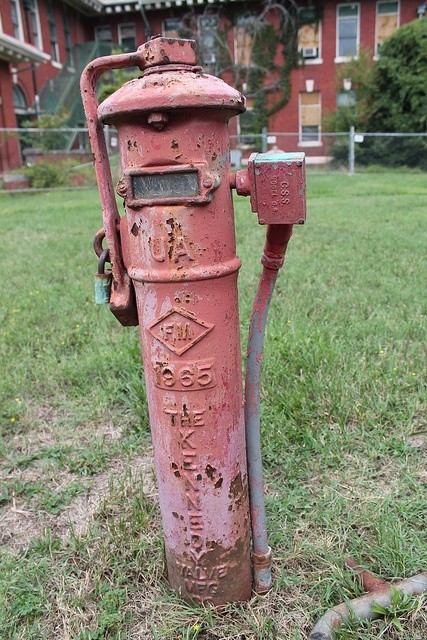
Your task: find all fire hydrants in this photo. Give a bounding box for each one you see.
[79,37,307,606]
